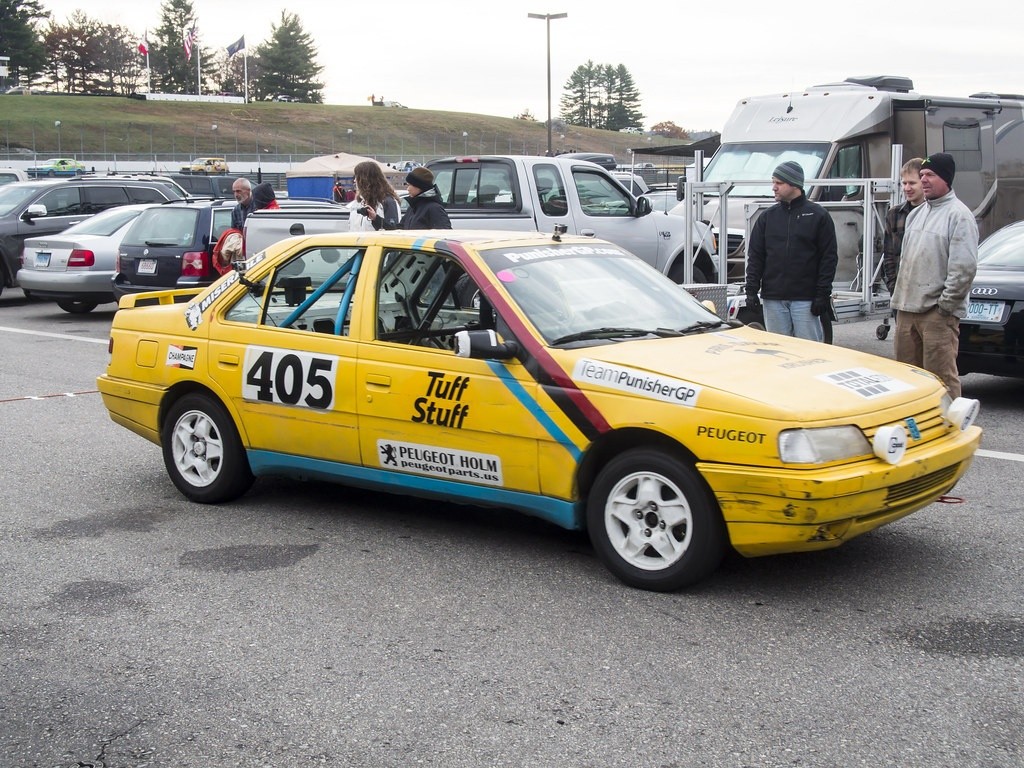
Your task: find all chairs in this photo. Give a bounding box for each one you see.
[538,177,553,206]
[471,185,500,202]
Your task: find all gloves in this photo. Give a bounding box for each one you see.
[746,293,760,313]
[810,298,828,317]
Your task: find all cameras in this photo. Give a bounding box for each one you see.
[357,207,369,216]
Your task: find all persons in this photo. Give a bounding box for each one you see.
[889,153,978,402]
[346,162,401,272]
[229,177,258,231]
[251,182,279,211]
[746,162,837,344]
[882,158,924,314]
[366,165,452,230]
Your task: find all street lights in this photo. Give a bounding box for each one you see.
[527,13,570,155]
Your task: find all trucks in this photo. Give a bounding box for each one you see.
[669,78,1024,293]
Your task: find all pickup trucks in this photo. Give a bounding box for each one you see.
[243,155,721,286]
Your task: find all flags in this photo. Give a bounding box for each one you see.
[226,35,245,58]
[184,20,199,62]
[138,28,149,56]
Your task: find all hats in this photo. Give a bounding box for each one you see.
[773,160,804,190]
[406,168,434,192]
[919,153,955,188]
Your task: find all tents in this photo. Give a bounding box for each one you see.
[286,152,401,205]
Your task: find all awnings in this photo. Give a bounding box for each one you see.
[627,134,721,209]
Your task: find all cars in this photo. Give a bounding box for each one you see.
[116,195,362,308]
[87,223,988,592]
[25,158,86,177]
[17,204,182,312]
[391,160,422,171]
[177,157,229,177]
[1,176,191,303]
[954,219,1024,378]
